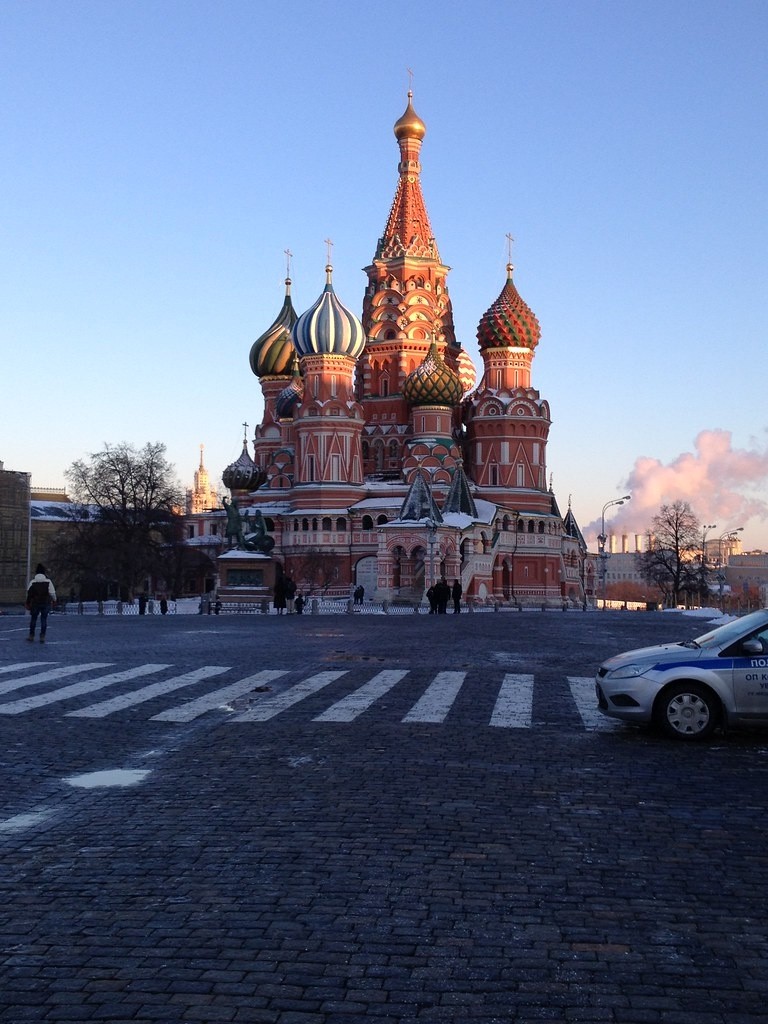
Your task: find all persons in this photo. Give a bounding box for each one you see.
[273,578,287,615]
[426,580,451,614]
[139,593,148,615]
[222,496,248,550]
[354,585,364,605]
[245,510,267,543]
[452,579,462,614]
[25,563,57,642]
[295,594,305,615]
[161,596,167,615]
[215,596,221,615]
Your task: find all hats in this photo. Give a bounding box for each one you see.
[35,563,47,574]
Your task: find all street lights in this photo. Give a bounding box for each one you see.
[601,495,631,610]
[16,472,32,616]
[701,524,744,596]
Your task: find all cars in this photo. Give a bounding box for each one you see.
[595,607,768,744]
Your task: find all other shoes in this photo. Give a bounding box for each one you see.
[40,636,45,642]
[26,636,33,641]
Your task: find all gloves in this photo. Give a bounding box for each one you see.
[51,600,56,606]
[25,603,30,610]
